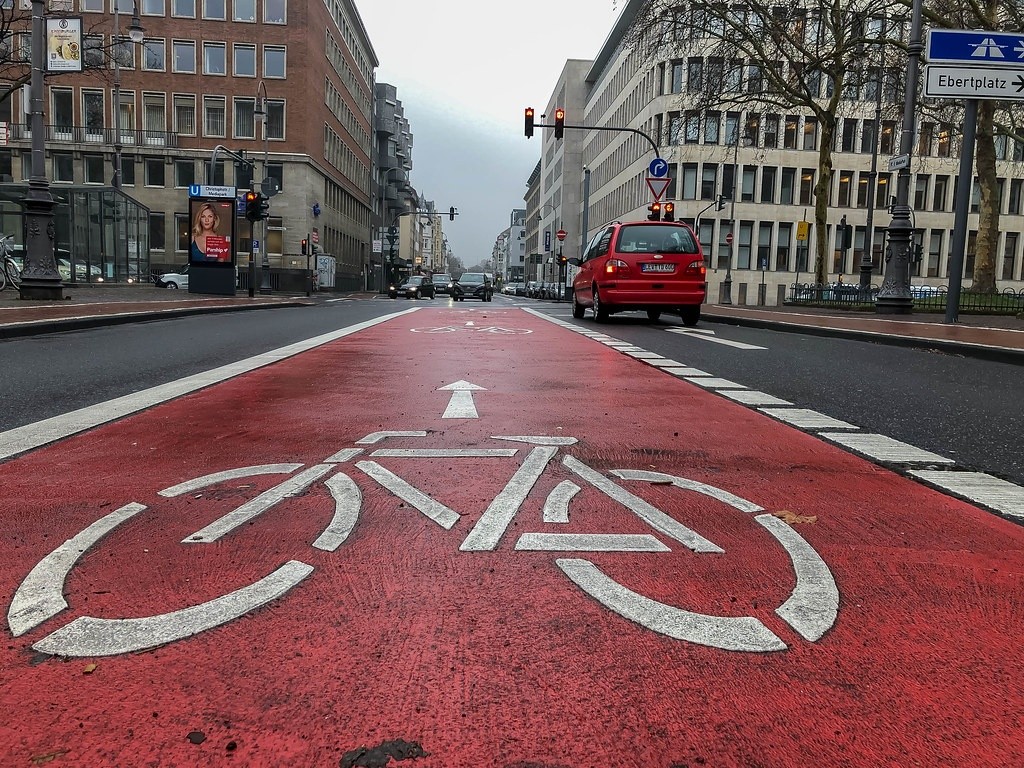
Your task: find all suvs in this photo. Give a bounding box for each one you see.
[568,220,707,326]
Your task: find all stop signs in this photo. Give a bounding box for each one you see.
[557,230,566,239]
[726,234,733,244]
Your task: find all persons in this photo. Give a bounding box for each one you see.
[388,267,395,284]
[192,203,225,262]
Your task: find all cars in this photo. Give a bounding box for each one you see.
[154,262,239,290]
[798,283,941,302]
[0,244,142,282]
[388,273,495,302]
[501,281,565,299]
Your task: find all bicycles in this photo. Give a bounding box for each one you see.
[0,234,22,292]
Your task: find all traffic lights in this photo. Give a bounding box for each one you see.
[245,191,270,222]
[664,202,674,222]
[555,108,564,140]
[715,195,727,211]
[647,203,660,221]
[302,240,318,255]
[556,254,566,266]
[525,107,534,139]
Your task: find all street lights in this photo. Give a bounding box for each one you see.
[536,205,557,289]
[114,0,152,283]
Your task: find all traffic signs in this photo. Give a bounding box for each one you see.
[923,64,1024,100]
